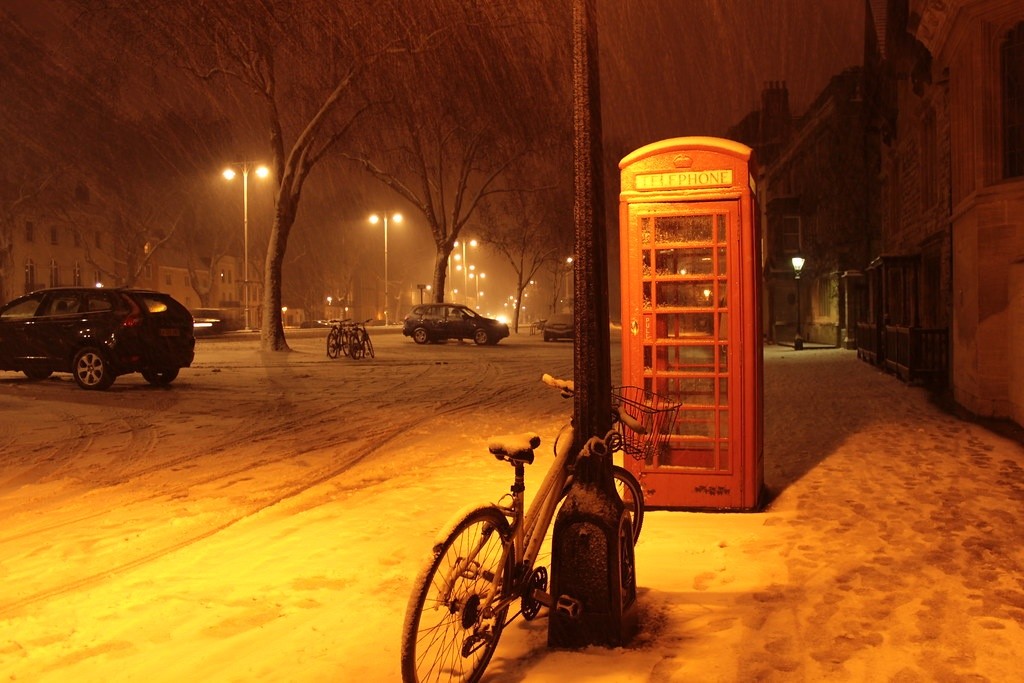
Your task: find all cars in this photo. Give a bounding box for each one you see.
[543,313,575,341]
[299,320,344,329]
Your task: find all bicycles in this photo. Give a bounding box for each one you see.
[400,371,683,683]
[326,319,374,360]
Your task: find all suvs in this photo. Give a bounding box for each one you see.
[0,286,194,391]
[403,304,509,345]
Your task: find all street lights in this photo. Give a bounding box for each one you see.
[368,211,402,326]
[222,161,268,334]
[789,253,805,352]
